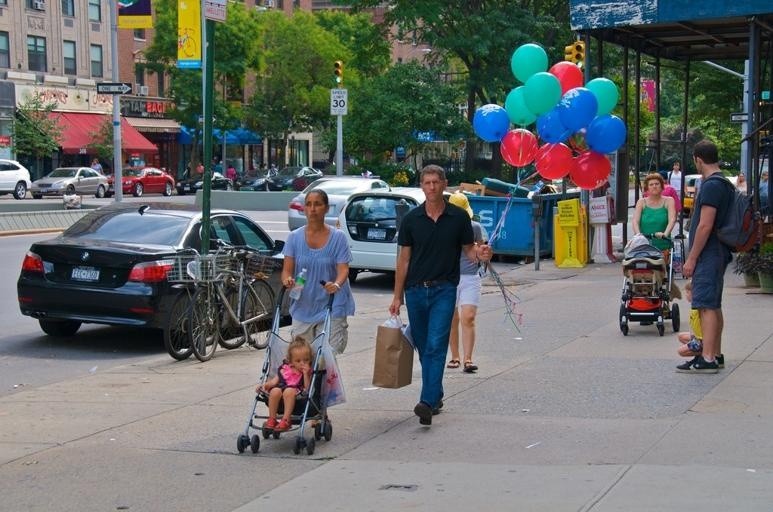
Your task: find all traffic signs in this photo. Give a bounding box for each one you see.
[329,88,347,117]
[197,114,216,126]
[96,82,132,95]
[728,111,755,123]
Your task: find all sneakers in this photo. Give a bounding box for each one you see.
[414,402,432,428]
[638,321,655,326]
[432,398,445,414]
[262,418,277,428]
[675,355,719,374]
[716,352,726,369]
[275,419,294,432]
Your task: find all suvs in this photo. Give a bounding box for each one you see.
[0,159,35,200]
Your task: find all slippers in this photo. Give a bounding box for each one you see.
[447,358,461,369]
[463,362,479,373]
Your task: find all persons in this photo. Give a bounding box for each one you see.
[181,156,223,177]
[757,170,769,207]
[632,172,677,327]
[90,158,104,175]
[279,187,357,428]
[386,163,495,425]
[673,139,733,376]
[735,171,747,194]
[692,175,705,205]
[676,282,705,357]
[225,165,238,180]
[445,191,485,373]
[267,163,279,178]
[667,159,688,200]
[254,335,314,431]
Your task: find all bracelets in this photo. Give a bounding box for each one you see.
[333,282,340,288]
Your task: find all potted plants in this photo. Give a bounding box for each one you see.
[731,250,758,287]
[754,241,772,291]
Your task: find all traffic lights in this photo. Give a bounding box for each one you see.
[571,40,585,72]
[564,42,573,62]
[332,59,343,84]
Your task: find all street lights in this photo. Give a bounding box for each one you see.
[404,46,430,65]
[254,5,266,14]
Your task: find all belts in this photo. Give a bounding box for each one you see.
[406,276,458,288]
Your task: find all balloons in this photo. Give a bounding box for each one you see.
[469,43,630,192]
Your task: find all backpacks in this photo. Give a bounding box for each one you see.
[698,176,760,253]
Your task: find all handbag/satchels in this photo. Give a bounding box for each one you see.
[372,315,413,389]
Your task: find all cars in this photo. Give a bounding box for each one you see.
[283,174,392,233]
[29,164,110,201]
[350,161,415,178]
[683,172,703,214]
[329,184,458,285]
[237,165,278,192]
[173,169,234,196]
[103,164,178,198]
[14,201,290,353]
[265,165,323,192]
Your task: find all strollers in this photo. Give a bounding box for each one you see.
[229,277,338,460]
[615,231,682,340]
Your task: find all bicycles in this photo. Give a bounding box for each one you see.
[183,244,278,365]
[158,236,256,364]
